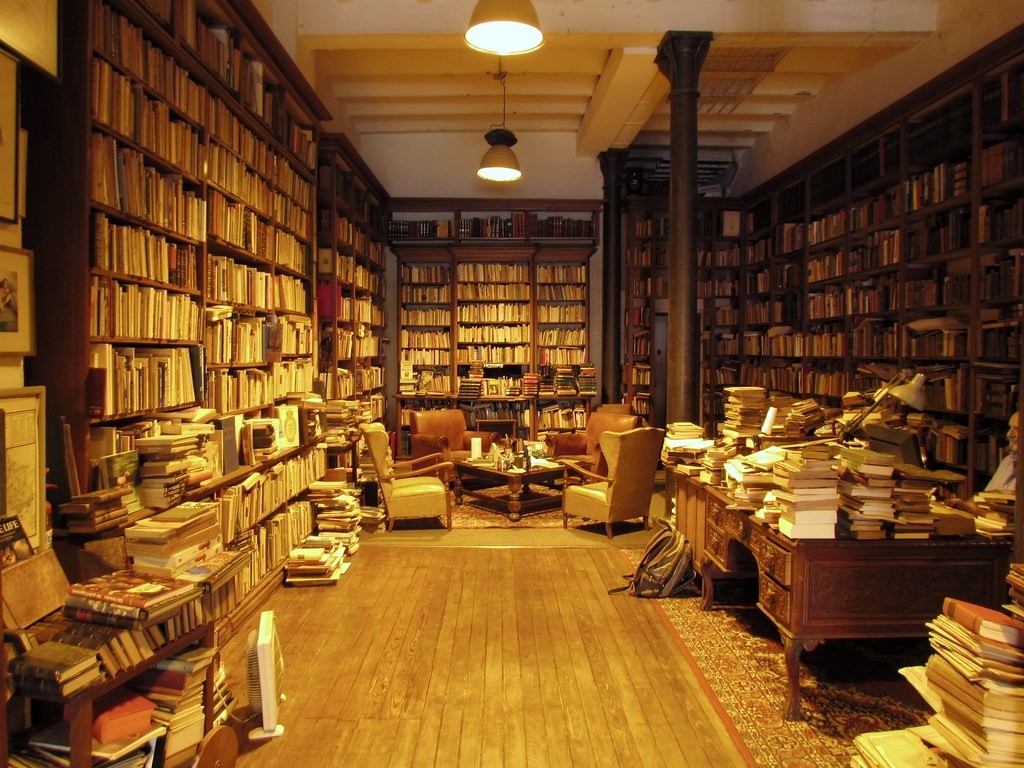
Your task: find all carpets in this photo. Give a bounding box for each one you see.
[618,546,942,768]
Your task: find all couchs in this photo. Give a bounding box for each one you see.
[546,411,639,493]
[408,408,502,492]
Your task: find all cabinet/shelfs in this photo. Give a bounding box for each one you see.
[318,135,391,532]
[35,0,325,626]
[391,197,606,462]
[615,194,744,426]
[745,24,1024,500]
[18,619,216,768]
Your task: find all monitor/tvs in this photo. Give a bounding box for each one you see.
[865,423,924,469]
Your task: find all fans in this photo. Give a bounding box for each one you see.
[244,610,287,740]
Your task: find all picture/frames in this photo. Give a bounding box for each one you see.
[0,48,21,224]
[0,244,36,358]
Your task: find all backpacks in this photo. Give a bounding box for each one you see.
[607,517,692,600]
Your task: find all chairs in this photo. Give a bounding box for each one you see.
[562,426,665,538]
[358,421,462,531]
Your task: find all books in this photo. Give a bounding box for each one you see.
[622,64,1024,768]
[389,214,597,458]
[0,1,386,768]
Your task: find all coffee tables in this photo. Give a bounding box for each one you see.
[454,457,595,520]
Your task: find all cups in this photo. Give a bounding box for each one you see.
[505,443,512,457]
[497,460,511,471]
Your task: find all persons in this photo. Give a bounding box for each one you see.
[983,411,1019,491]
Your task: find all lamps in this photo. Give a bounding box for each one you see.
[836,370,926,443]
[464,0,545,54]
[477,72,522,182]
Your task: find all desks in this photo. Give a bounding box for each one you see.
[662,466,707,575]
[703,485,1012,723]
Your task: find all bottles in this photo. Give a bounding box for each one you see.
[518,438,523,452]
[489,443,499,461]
[514,451,524,468]
[498,452,508,460]
